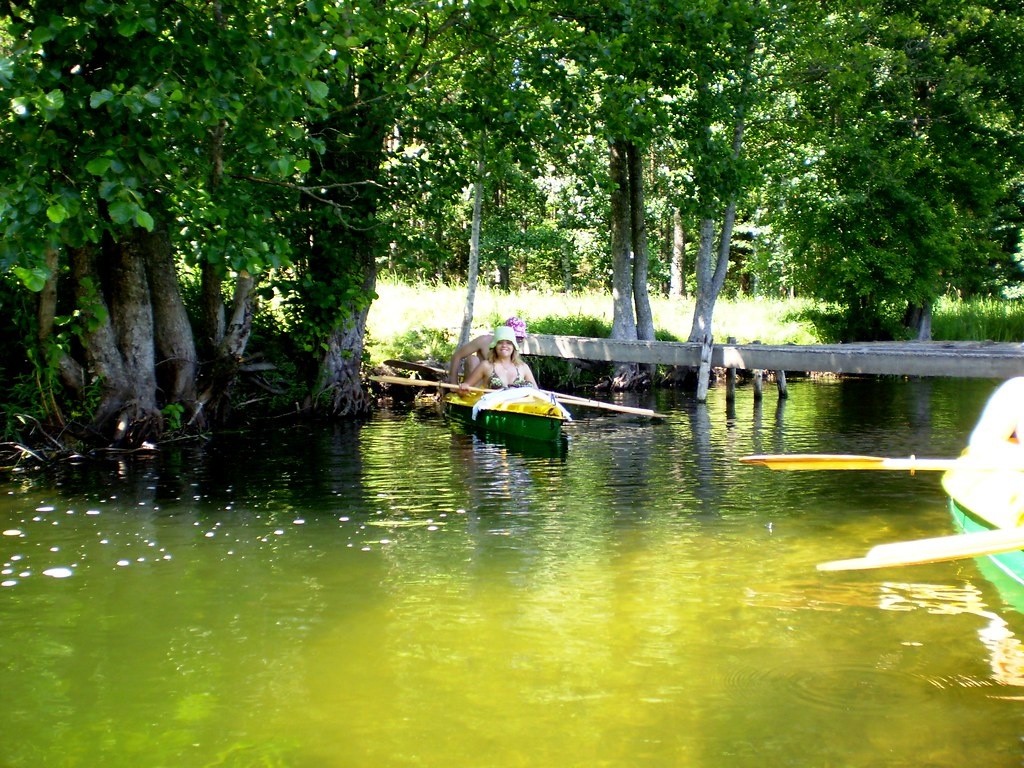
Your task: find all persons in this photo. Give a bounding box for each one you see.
[449,316,528,393]
[456,325,539,396]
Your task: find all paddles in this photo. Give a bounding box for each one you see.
[813,525,1024,575]
[366,373,654,417]
[382,356,672,419]
[740,447,954,475]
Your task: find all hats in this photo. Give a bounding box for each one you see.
[504,316,527,338]
[489,326,519,351]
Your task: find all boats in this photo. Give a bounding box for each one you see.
[941,437,1024,616]
[441,385,566,441]
